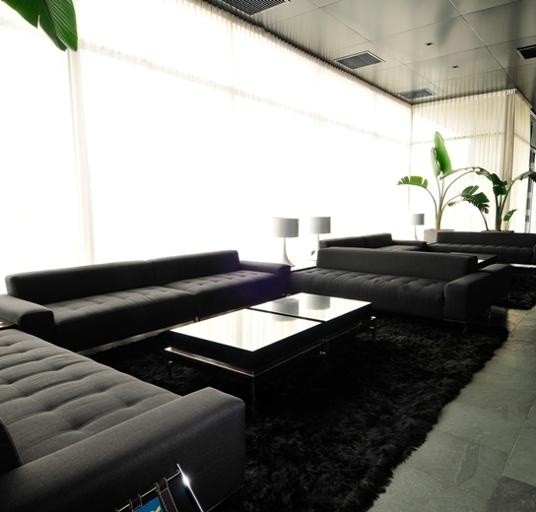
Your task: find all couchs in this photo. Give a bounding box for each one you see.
[320,232,536,304]
[290,246,493,330]
[0,326,250,512]
[1,250,289,366]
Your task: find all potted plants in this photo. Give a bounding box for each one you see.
[395,129,536,245]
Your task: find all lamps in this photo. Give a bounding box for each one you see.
[272,215,331,266]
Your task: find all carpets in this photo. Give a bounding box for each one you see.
[102,292,510,511]
[490,265,536,310]
